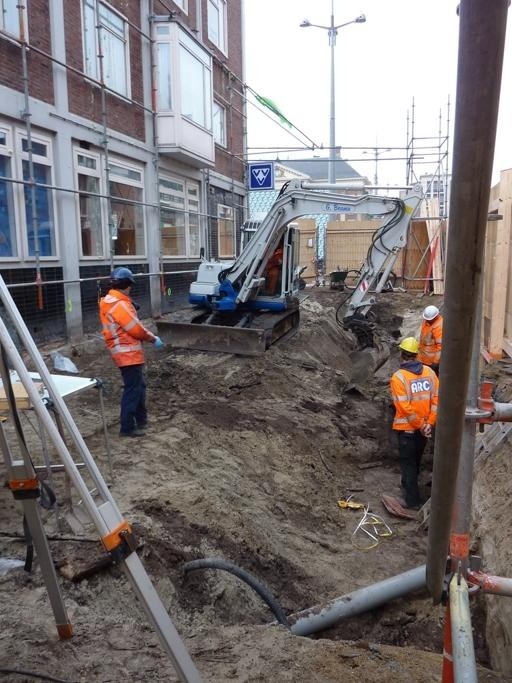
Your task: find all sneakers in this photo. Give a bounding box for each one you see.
[118,425,153,438]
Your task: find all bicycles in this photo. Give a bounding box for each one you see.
[343,255,398,292]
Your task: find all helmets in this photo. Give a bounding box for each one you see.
[112,267,136,283]
[423,305,440,320]
[399,337,419,354]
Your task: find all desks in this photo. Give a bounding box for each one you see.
[4,369,114,520]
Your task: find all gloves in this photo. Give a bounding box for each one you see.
[153,336,165,349]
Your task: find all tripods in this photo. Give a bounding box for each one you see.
[0,275,204,683]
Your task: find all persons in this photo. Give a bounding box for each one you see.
[259,238,283,294]
[389,334,440,512]
[412,304,444,376]
[99,266,166,437]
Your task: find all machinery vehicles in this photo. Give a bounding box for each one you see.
[154,179,427,396]
